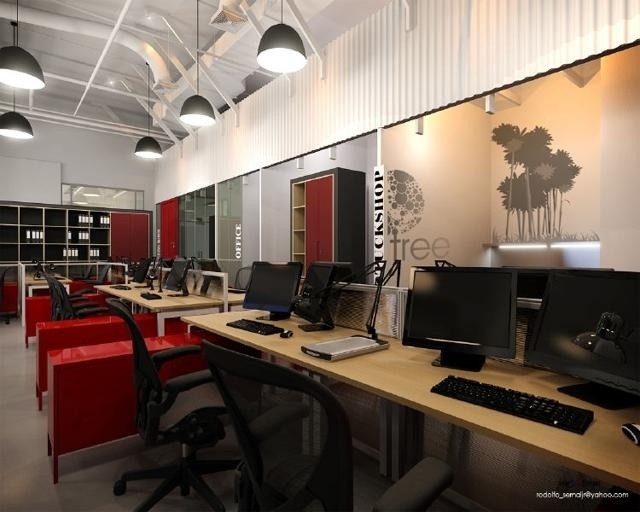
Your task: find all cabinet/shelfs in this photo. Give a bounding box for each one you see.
[288,167,367,281]
[0,200,154,318]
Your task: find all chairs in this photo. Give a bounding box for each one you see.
[38,271,110,322]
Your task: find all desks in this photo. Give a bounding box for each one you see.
[24,272,73,298]
[94,280,245,340]
[179,307,614,510]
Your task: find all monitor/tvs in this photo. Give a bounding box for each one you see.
[525,270,640,410]
[242,261,304,321]
[517,269,614,299]
[107,256,221,297]
[294,263,337,332]
[401,265,518,372]
[332,262,357,283]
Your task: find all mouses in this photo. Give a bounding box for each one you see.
[280,330,292,339]
[621,423,640,446]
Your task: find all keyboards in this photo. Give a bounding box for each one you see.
[226,318,284,336]
[430,375,593,435]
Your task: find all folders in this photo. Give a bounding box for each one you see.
[61,214,111,260]
[25,229,43,243]
